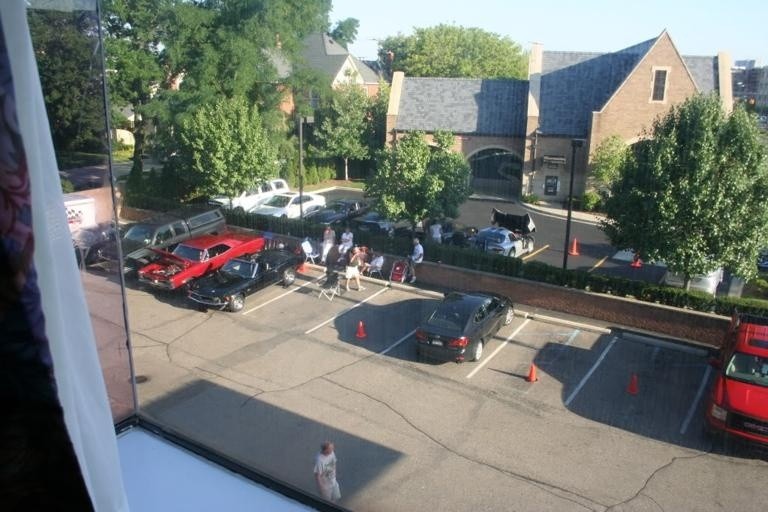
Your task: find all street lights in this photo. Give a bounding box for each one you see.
[297,115,315,219]
[562,138,587,270]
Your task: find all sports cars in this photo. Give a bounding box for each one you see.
[463,207,537,260]
[180,245,306,314]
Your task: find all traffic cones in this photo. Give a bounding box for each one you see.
[630,254,642,268]
[355,320,367,338]
[526,364,539,383]
[624,373,641,396]
[567,237,580,256]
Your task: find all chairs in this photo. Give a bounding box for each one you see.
[299,236,383,302]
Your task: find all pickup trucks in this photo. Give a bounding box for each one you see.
[95,200,231,279]
[706,306,768,449]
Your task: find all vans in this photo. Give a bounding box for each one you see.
[215,178,291,215]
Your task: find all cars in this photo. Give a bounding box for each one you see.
[303,195,369,235]
[414,288,514,364]
[660,263,723,297]
[66,217,137,273]
[348,207,412,238]
[136,225,266,296]
[250,189,326,223]
[60,167,105,194]
[405,217,479,246]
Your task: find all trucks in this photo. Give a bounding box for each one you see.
[62,193,96,235]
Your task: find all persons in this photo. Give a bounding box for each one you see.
[429,218,443,244]
[313,442,341,503]
[408,238,424,284]
[321,226,383,292]
